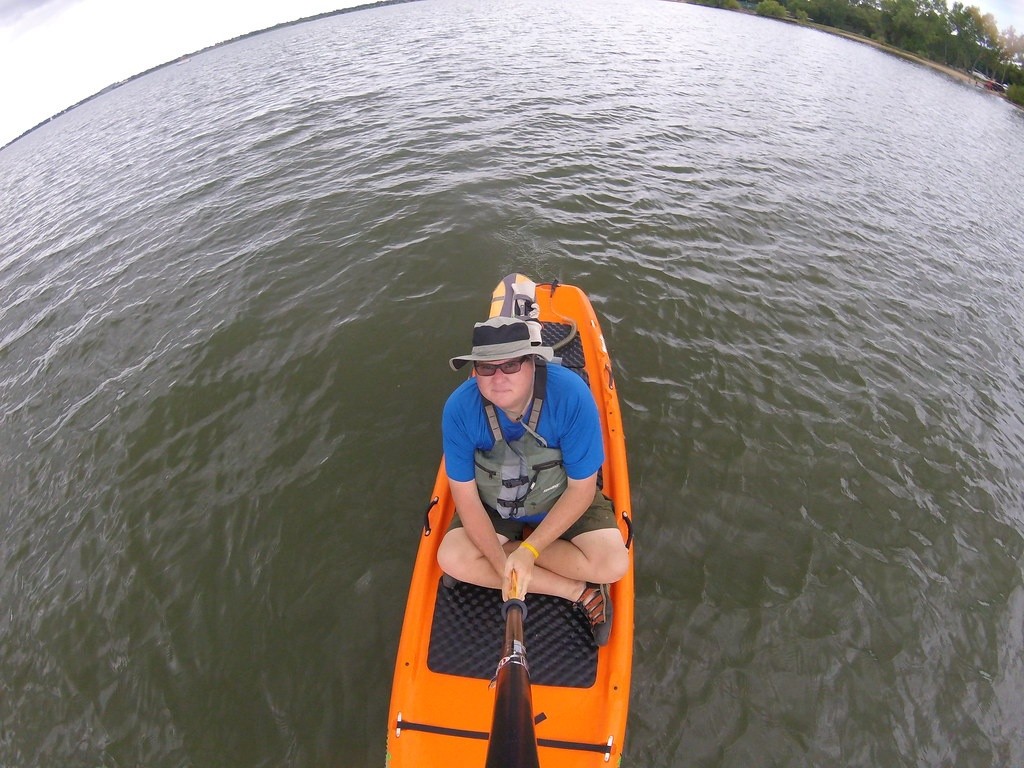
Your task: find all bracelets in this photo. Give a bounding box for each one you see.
[520,541,539,559]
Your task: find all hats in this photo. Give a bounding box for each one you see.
[449,317,554,372]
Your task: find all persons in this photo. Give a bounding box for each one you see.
[436,316,629,648]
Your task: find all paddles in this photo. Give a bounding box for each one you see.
[485,571,540,768]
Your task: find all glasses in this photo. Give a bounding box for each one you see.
[474,356,527,376]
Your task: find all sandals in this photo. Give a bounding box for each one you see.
[443,572,457,588]
[576,582,611,646]
